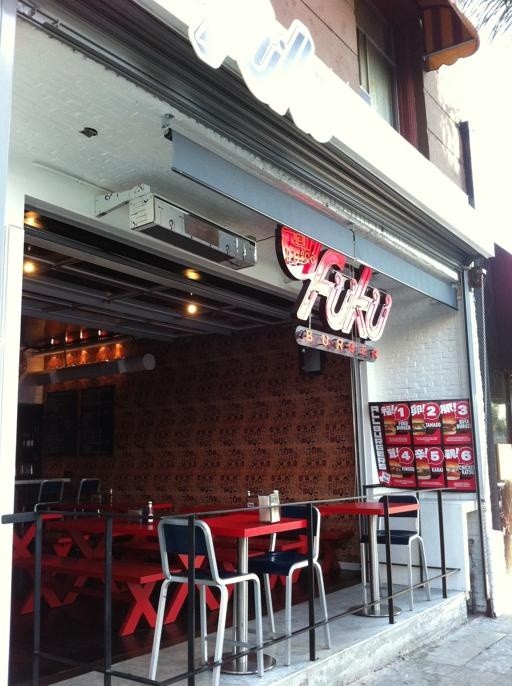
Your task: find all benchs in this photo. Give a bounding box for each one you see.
[112,542,265,600]
[13,558,181,637]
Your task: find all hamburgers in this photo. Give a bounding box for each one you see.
[388,460,403,478]
[442,420,456,435]
[446,460,461,480]
[411,419,426,436]
[415,461,432,480]
[383,421,397,435]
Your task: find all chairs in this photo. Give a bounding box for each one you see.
[21,479,64,539]
[74,478,102,519]
[233,504,330,666]
[149,517,264,686]
[360,494,431,611]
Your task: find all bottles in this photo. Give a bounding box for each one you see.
[100,486,114,504]
[146,501,155,525]
[246,491,254,511]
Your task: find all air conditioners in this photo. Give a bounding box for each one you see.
[129,192,258,271]
